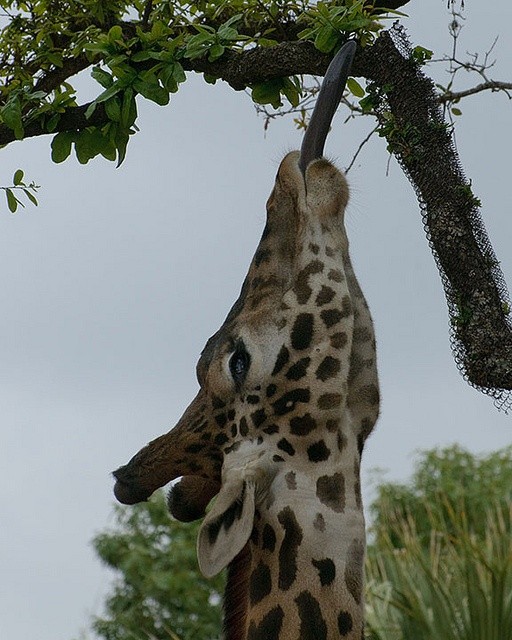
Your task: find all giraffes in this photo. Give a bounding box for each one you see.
[111,40,380,640]
